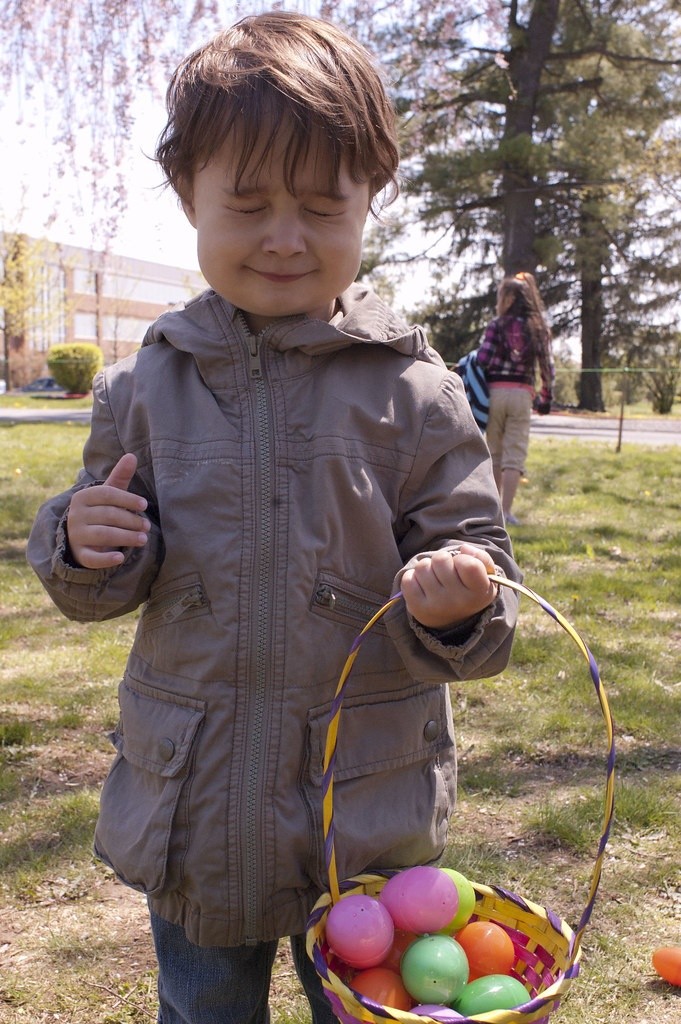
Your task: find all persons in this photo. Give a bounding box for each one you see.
[473,270,556,526]
[23,10,517,1024]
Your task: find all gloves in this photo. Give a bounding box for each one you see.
[533,393,552,417]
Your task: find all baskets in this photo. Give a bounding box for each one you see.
[303,571,614,1024]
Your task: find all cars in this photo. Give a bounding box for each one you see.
[20,375,64,394]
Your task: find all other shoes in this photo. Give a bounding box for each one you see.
[504,514,524,527]
